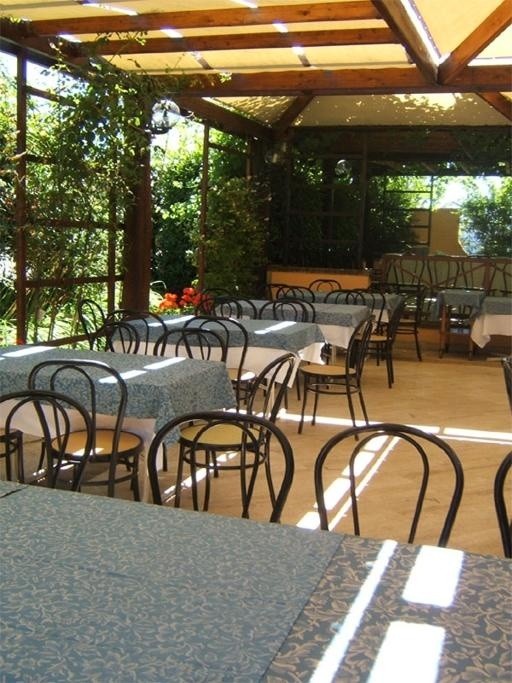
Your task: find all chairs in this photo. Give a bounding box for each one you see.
[498,352,512,411]
[399,249,511,363]
[315,421,467,550]
[172,351,298,518]
[143,409,297,530]
[351,304,396,391]
[0,390,95,493]
[78,299,106,343]
[88,319,151,354]
[192,276,397,328]
[181,315,255,407]
[298,315,376,442]
[151,325,228,363]
[495,445,511,561]
[26,358,144,502]
[108,308,169,357]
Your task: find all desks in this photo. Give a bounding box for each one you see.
[113,314,326,413]
[0,343,233,477]
[0,477,511,683]
